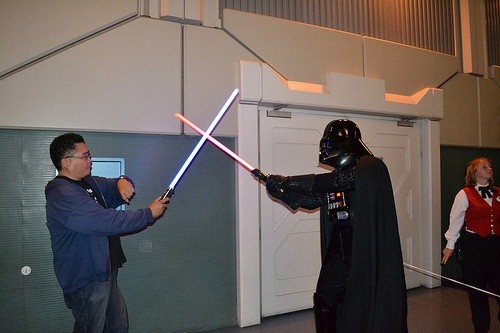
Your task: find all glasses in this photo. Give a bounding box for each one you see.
[64,153,91,160]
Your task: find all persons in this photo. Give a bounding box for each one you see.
[266,119,409,333]
[45,133,169,333]
[441,158,500,333]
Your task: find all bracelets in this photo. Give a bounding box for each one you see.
[120,175,127,179]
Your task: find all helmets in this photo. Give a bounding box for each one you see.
[319,118,361,167]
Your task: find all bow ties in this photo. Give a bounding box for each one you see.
[478,185,493,199]
